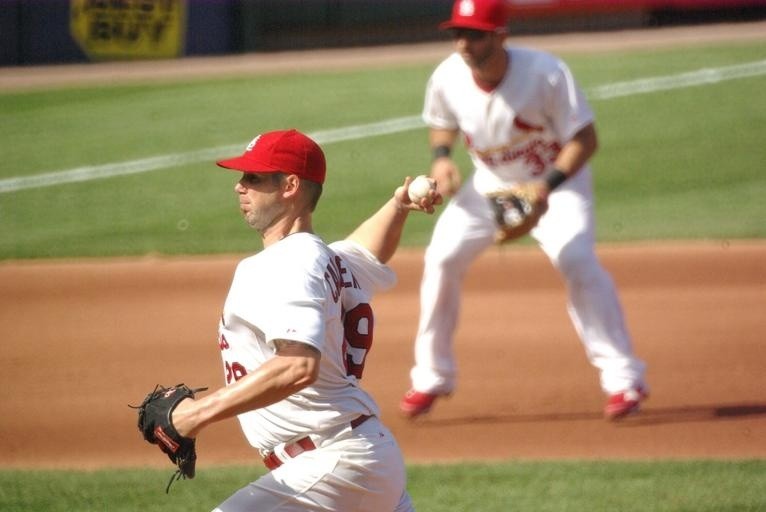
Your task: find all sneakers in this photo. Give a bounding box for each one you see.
[605,386,646,420]
[399,388,437,413]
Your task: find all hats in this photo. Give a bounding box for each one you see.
[216,128,326,184]
[439,0,512,31]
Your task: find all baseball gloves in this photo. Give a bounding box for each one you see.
[137,383,197,479]
[487,179,549,242]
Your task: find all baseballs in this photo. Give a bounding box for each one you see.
[408,175,436,207]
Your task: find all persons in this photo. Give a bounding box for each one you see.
[399,0,649,422]
[129,128,442,512]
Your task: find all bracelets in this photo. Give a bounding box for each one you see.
[431,145,450,161]
[541,166,566,187]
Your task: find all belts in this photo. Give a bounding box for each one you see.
[263,415,370,470]
